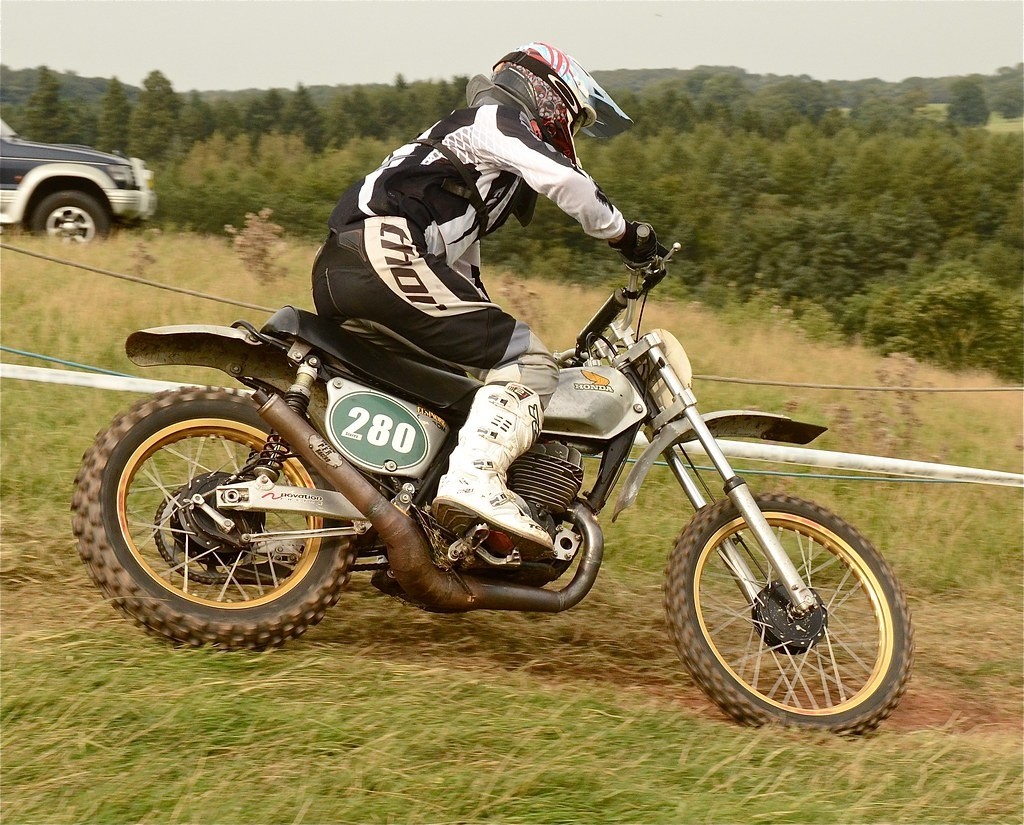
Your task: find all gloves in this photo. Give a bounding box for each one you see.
[607,219,670,265]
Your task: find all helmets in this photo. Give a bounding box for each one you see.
[489,42,598,141]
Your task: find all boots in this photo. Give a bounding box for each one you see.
[432,381,552,560]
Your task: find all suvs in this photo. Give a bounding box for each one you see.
[0,117,159,249]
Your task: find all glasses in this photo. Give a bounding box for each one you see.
[571,110,588,140]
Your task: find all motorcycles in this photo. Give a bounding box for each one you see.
[68,222,917,744]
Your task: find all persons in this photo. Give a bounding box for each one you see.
[310,41,675,562]
